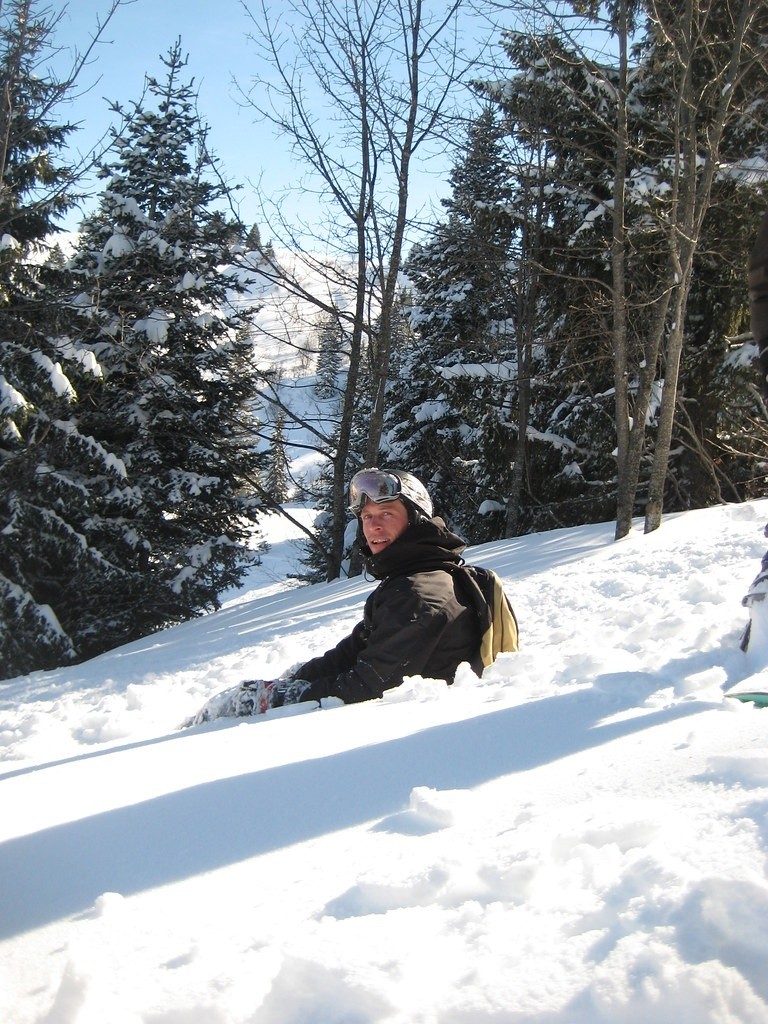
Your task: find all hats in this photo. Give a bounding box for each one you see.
[355,469,433,522]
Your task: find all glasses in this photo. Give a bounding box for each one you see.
[346,468,402,512]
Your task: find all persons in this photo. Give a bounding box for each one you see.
[175,469,485,728]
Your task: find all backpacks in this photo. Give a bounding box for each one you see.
[465,566,521,676]
